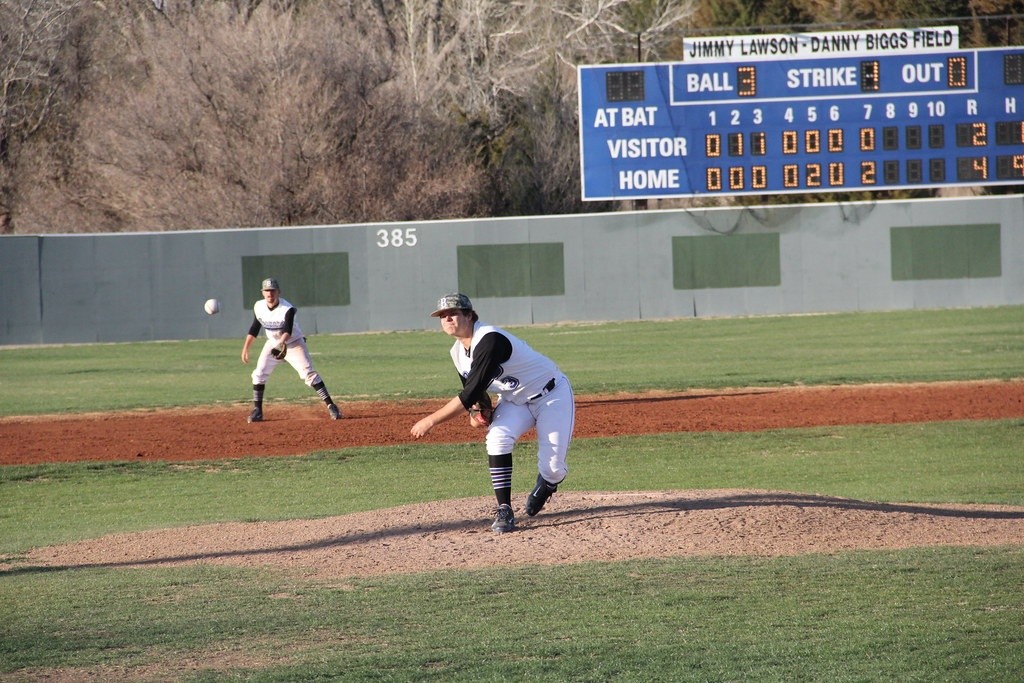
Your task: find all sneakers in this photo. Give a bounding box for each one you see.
[328,404,341,420]
[246,407,262,423]
[491,504,514,531]
[526,472,558,515]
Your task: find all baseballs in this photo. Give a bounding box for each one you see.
[204,298,220,316]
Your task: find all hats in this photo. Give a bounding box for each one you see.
[429,292,472,317]
[260,278,279,291]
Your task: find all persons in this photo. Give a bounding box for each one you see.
[242,279,340,422]
[410,294,575,532]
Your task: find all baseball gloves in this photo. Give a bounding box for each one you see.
[469,392,493,429]
[270,342,287,360]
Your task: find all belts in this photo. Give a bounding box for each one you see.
[531,378,555,400]
